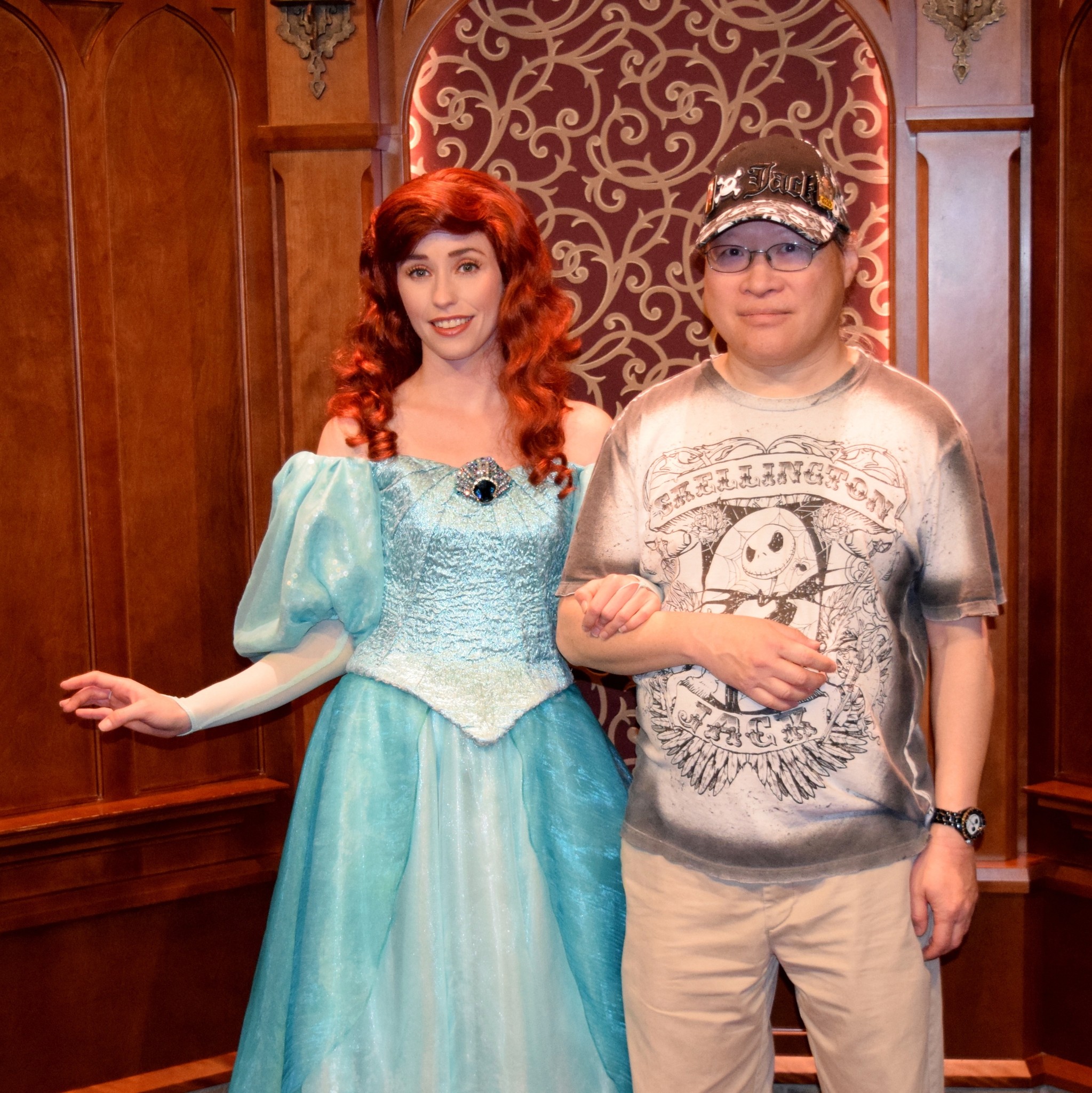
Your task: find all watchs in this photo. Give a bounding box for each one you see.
[931,805,987,845]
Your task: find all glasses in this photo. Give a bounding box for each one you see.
[699,238,834,273]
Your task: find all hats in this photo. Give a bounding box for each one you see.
[695,136,852,247]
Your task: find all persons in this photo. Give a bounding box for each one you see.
[553,140,1004,1093]
[54,167,669,1093]
